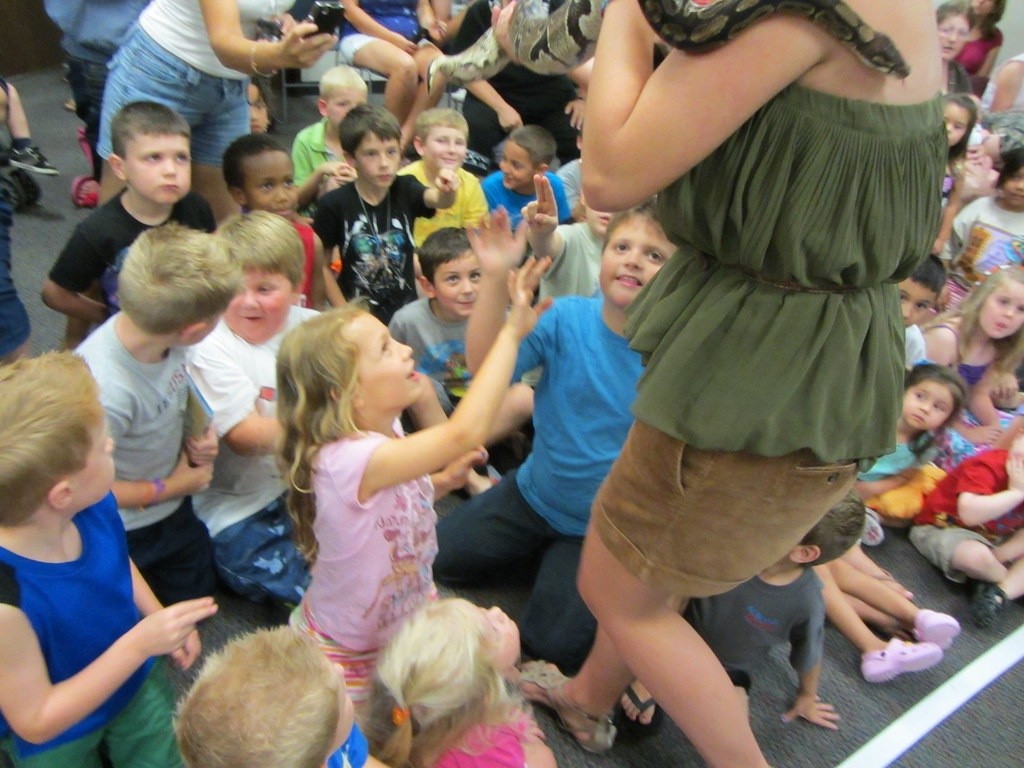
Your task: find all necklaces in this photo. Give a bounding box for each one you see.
[357,184,391,257]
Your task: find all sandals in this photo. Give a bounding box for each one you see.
[860,637,943,684]
[503,660,617,753]
[975,581,1010,627]
[914,609,960,649]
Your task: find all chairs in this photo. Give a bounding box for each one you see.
[282,0,458,123]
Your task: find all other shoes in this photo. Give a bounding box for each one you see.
[62,74,72,82]
[862,508,884,546]
[66,100,76,110]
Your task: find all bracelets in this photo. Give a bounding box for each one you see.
[249,41,277,77]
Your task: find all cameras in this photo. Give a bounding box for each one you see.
[303,0,345,37]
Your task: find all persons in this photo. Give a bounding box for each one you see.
[682,0,1024,727]
[517,0,948,768]
[0,0,677,768]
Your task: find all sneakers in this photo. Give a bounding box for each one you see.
[9,148,59,175]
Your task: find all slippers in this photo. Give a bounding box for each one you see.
[79,127,92,167]
[72,175,99,208]
[617,686,661,733]
[873,568,896,582]
